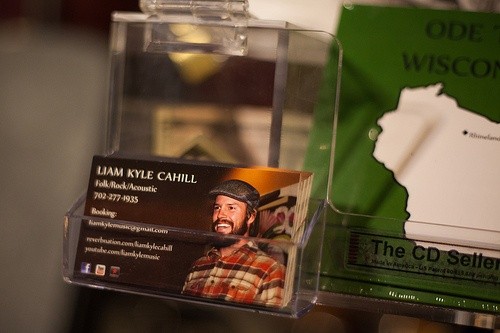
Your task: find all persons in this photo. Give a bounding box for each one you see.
[178,179,285,308]
